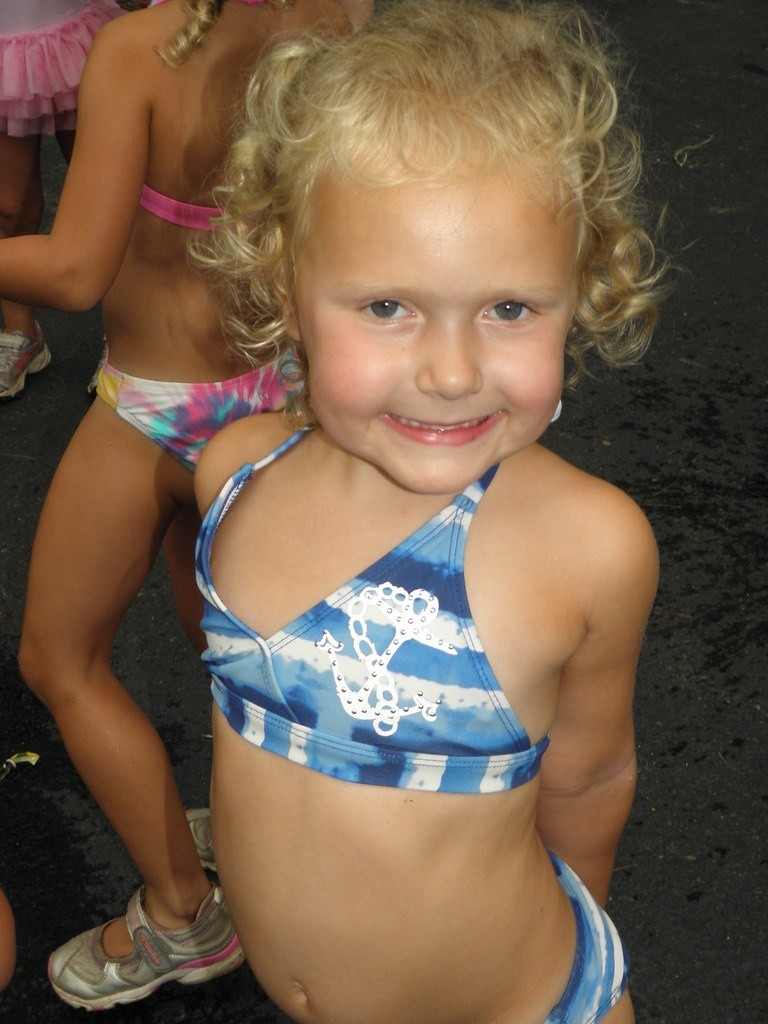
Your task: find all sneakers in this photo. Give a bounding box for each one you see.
[0,318,50,395]
[48,877,244,1009]
[184,805,218,875]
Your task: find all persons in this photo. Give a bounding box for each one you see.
[1,1,377,1011]
[1,0,138,399]
[183,0,660,1023]
